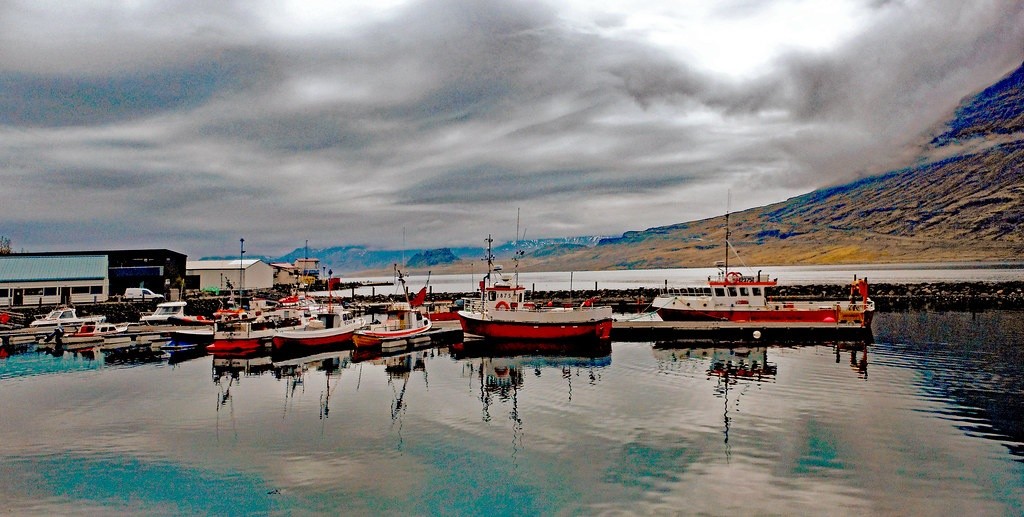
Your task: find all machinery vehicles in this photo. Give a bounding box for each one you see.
[295,269,328,292]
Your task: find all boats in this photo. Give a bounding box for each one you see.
[456,207,619,344]
[652,188,876,333]
[0,237,654,361]
[351,226,434,353]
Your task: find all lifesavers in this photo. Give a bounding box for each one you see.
[495,301,510,311]
[732,272,742,280]
[726,272,738,284]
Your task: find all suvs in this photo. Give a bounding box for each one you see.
[124,287,166,304]
